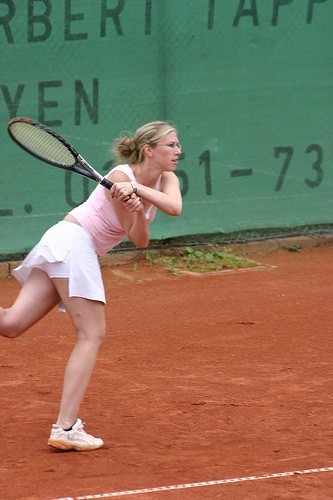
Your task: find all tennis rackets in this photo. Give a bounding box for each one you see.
[7,116,131,202]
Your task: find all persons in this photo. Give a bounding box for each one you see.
[0,121,184,453]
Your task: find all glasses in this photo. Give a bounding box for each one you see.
[152,143,182,150]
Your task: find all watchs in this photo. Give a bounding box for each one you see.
[129,181,138,193]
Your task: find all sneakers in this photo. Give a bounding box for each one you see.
[47,417,105,452]
